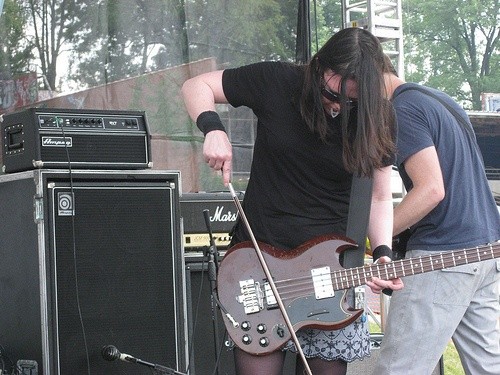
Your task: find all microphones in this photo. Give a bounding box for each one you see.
[100,344,185,375]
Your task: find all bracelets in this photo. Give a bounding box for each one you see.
[196,110,227,136]
[373,245,393,263]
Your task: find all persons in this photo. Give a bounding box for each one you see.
[372,52,500,375]
[181,27,405,375]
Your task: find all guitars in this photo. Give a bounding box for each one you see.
[217,233,500,355]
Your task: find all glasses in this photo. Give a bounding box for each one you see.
[319,70,358,106]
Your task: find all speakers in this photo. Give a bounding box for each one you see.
[0,170,187,375]
[189,260,300,375]
